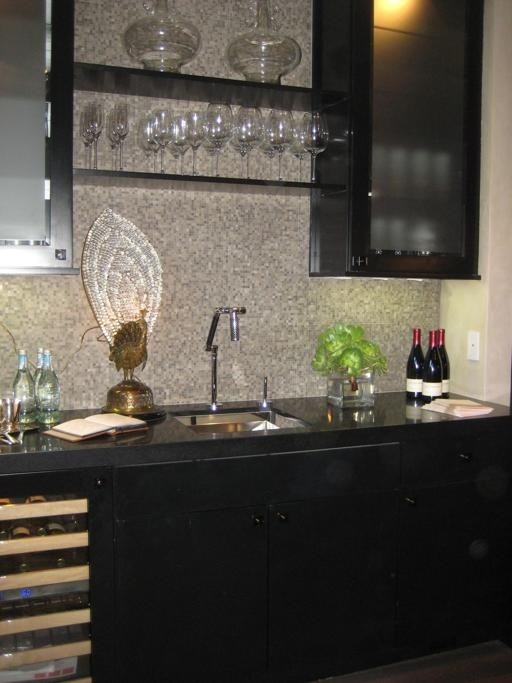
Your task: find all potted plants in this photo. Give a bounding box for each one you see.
[311,321,389,409]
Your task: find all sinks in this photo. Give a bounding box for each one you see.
[169,406,310,439]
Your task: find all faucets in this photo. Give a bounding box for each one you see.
[206,307,246,410]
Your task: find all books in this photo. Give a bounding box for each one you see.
[420,399,495,417]
[42,413,149,443]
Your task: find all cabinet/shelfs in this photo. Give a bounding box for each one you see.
[115,398,402,683]
[402,392,512,660]
[0,1,81,277]
[72,60,312,197]
[1,408,114,683]
[307,0,482,281]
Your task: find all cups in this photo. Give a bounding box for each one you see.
[232,106,263,178]
[136,120,151,171]
[207,101,231,175]
[144,117,158,171]
[233,136,246,163]
[84,111,93,169]
[106,115,114,170]
[178,145,190,175]
[154,111,174,173]
[185,111,204,174]
[266,106,291,177]
[204,140,215,176]
[110,110,120,171]
[292,119,307,182]
[115,102,131,170]
[88,101,103,171]
[79,111,89,168]
[260,116,274,180]
[171,117,186,147]
[303,112,327,183]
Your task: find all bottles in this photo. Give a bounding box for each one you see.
[405,327,424,399]
[0,492,89,577]
[422,330,443,403]
[32,347,44,398]
[36,349,61,425]
[13,349,36,424]
[437,328,451,399]
